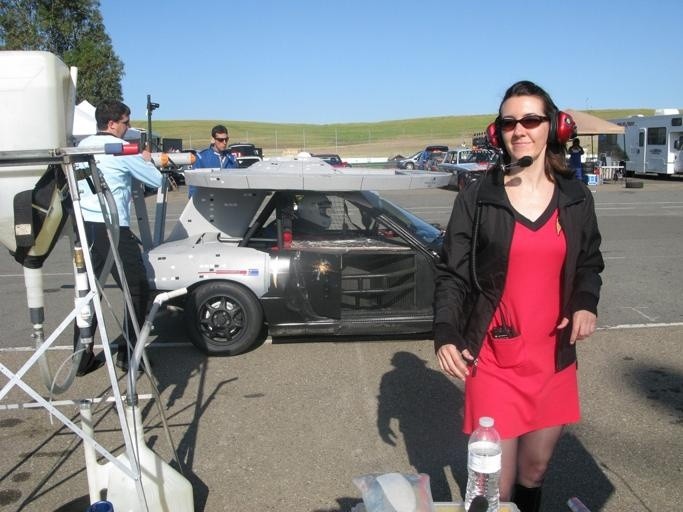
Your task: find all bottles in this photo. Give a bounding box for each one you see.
[462,416,501,511]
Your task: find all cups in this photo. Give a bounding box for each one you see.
[87,501,113,512]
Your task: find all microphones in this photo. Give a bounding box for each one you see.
[488,146,533,172]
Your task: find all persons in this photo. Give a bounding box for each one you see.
[70,99,162,377]
[567,138,584,179]
[296,193,333,239]
[185,125,239,201]
[432,80,603,510]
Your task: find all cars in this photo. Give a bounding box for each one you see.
[140,156,455,356]
[389,144,499,191]
[141,141,350,194]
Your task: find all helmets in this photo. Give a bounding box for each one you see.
[297,195,333,229]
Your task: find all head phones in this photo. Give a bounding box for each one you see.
[487,111,576,145]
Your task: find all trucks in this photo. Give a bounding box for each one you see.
[597,109,683,178]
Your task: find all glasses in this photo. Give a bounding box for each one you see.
[499,114,549,131]
[215,137,228,142]
